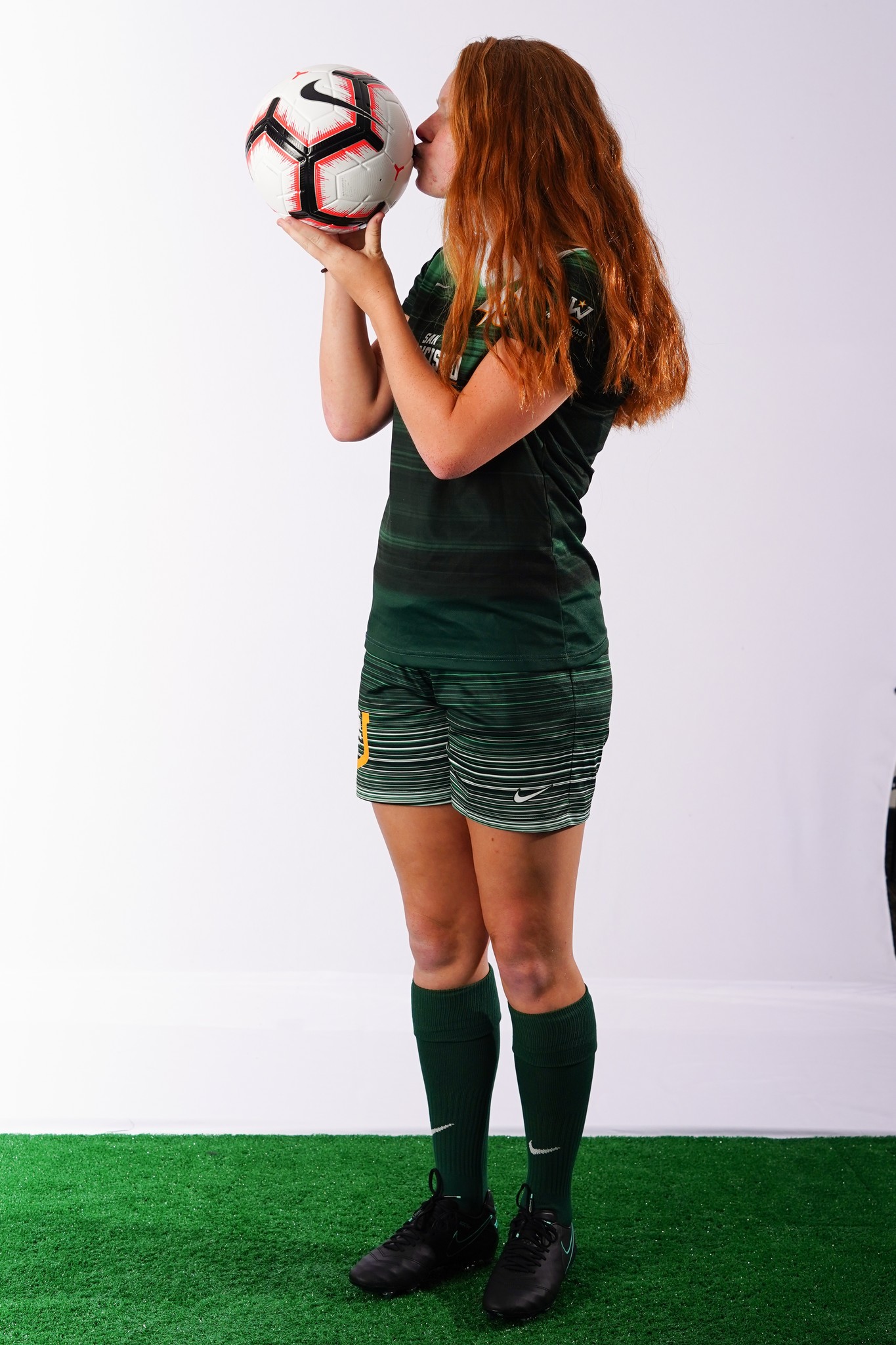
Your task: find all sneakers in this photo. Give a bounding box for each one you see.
[481,1181,578,1324]
[347,1168,501,1294]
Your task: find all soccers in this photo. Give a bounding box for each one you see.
[246,65,413,233]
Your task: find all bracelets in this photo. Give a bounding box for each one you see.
[321,267,328,273]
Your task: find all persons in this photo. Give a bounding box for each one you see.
[273,32,692,1323]
